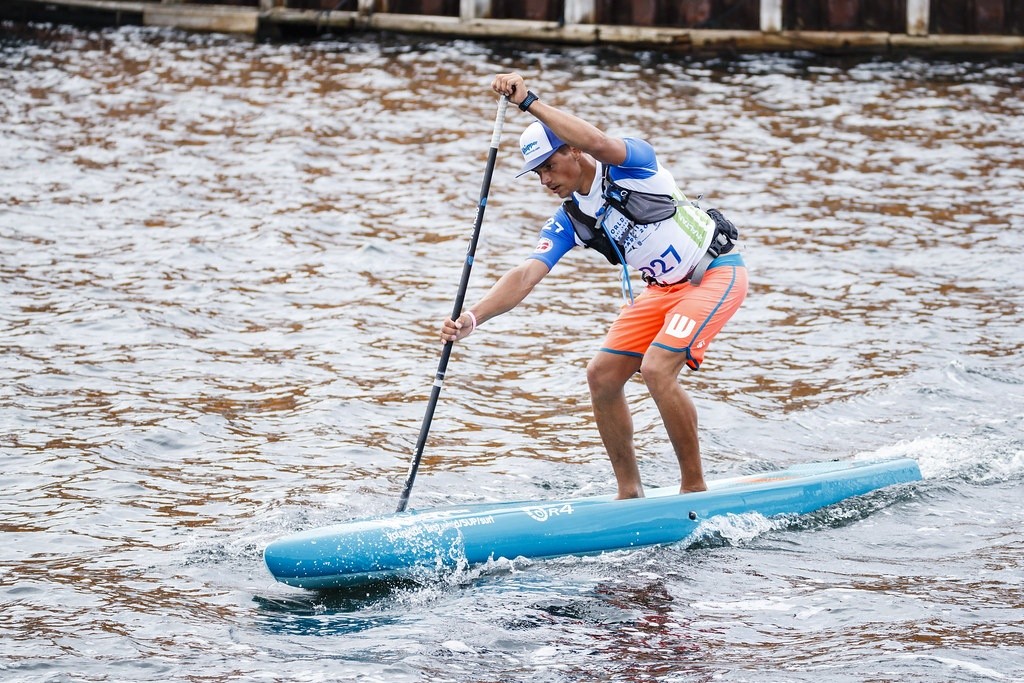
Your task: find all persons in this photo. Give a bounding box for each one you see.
[440,73,746,500]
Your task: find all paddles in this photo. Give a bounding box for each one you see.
[398,95,509,513]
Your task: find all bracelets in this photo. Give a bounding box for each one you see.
[463,310,476,337]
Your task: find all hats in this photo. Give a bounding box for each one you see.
[515,119,566,179]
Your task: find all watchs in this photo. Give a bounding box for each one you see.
[519,91,539,112]
